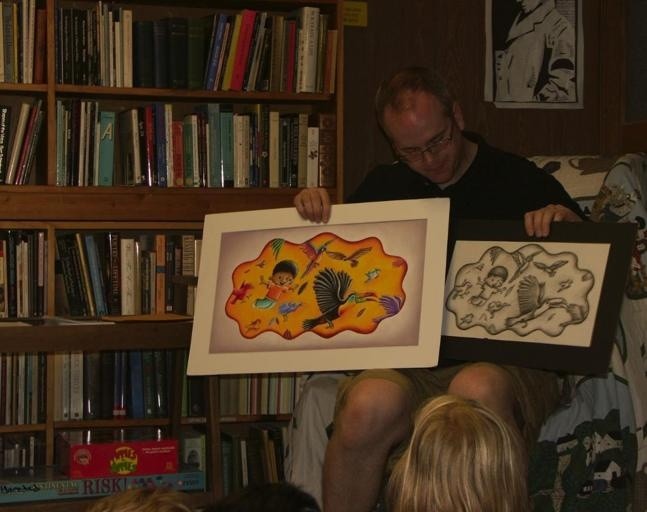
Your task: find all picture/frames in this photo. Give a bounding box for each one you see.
[186,196,451,375]
[436,217,638,378]
[484,0,584,109]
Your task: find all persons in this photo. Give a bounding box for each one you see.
[296,63,586,511]
[209,479,321,511]
[78,486,203,512]
[378,394,532,510]
[491,1,577,105]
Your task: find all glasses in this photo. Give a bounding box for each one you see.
[398,122,454,163]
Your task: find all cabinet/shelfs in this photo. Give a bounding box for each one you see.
[0,0,347,512]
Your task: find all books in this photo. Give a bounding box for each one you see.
[2,428,287,498]
[1,1,340,94]
[0,101,336,190]
[2,233,202,319]
[2,351,298,425]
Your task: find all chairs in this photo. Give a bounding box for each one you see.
[282,153,647,512]
